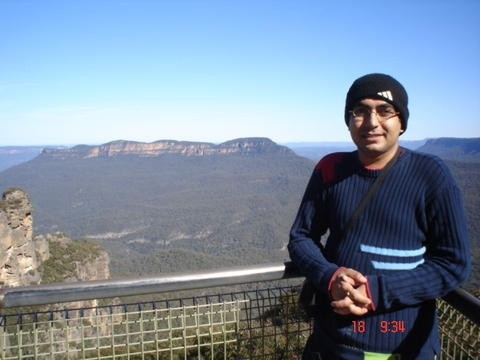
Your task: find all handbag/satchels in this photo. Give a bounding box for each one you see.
[297,259,320,323]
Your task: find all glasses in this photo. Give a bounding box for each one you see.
[348,105,403,122]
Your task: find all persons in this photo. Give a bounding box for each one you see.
[286,72,472,360]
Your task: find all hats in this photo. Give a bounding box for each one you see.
[344,73,411,136]
